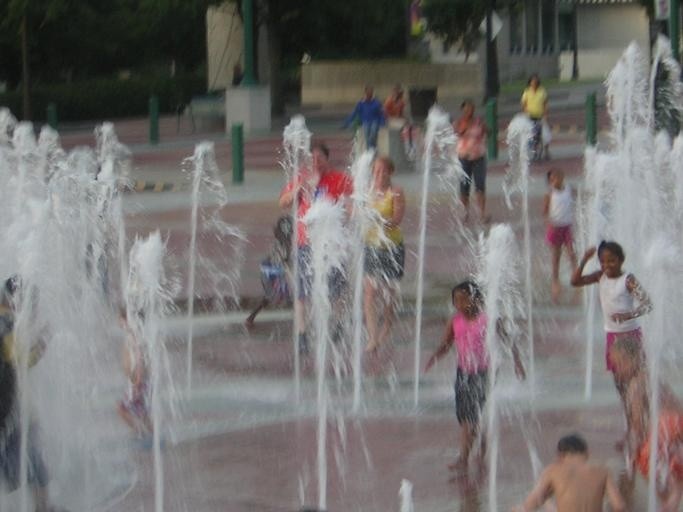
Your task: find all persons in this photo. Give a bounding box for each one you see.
[424,280,525,466]
[117,305,156,439]
[242,215,294,330]
[571,239,655,455]
[278,141,354,352]
[520,74,552,162]
[523,434,628,510]
[542,166,578,294]
[636,383,682,509]
[342,83,389,152]
[453,99,492,224]
[346,156,405,354]
[0,275,58,511]
[383,86,405,120]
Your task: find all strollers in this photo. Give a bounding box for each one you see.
[529,117,544,161]
